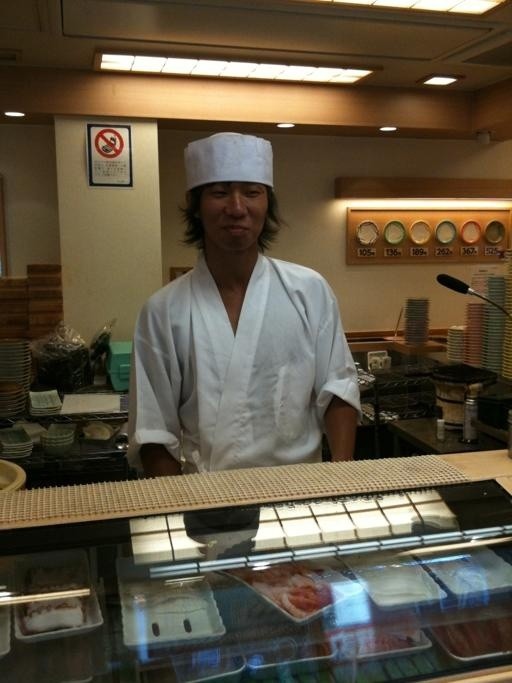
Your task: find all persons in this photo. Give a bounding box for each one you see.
[128,130,363,479]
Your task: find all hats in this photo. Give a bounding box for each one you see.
[178,132,275,193]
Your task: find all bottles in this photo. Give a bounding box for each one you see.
[463,398,480,445]
[436,419,445,441]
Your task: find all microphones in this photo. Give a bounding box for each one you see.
[437,274,512,322]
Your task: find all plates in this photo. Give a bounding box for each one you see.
[0,422,44,460]
[28,388,63,418]
[446,248,512,382]
[403,296,432,346]
[39,421,78,457]
[0,337,33,419]
